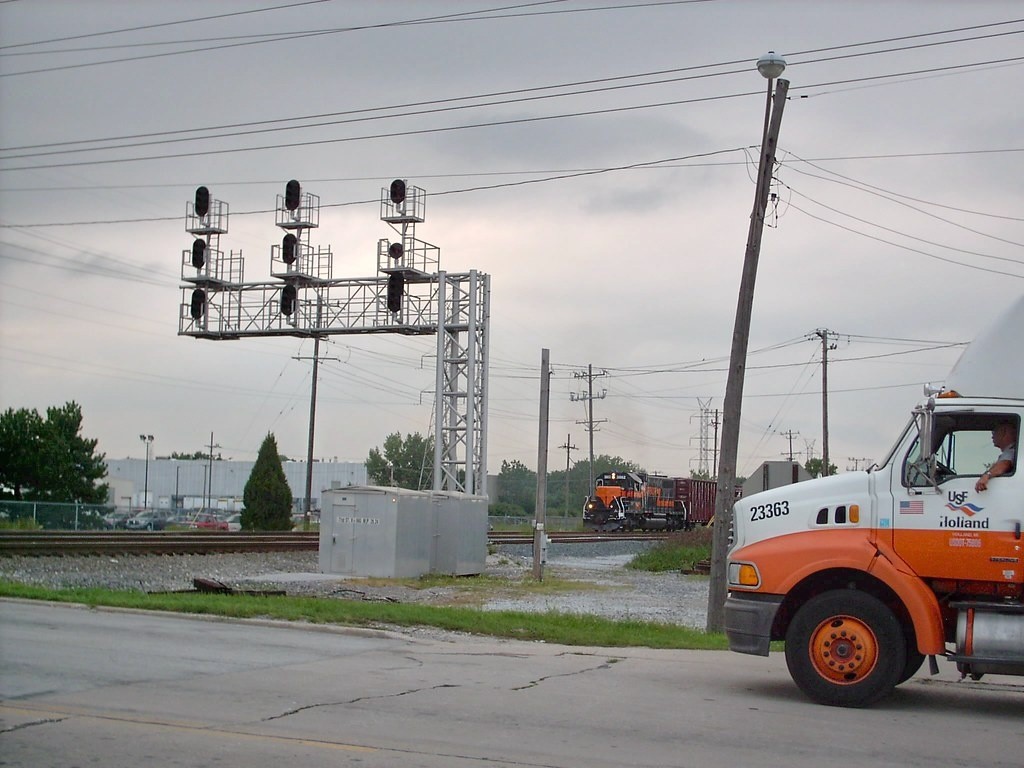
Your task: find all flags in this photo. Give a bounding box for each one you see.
[899,500,923,514]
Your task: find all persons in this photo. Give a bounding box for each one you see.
[975,420,1017,493]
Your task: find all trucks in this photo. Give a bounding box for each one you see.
[725,382,1023,709]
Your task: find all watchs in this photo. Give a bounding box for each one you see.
[984,471,991,478]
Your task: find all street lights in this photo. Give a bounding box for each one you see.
[139,434,157,511]
[705,50,790,635]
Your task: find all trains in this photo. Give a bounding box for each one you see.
[581,471,744,533]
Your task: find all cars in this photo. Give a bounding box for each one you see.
[93,509,244,532]
[289,513,319,533]
